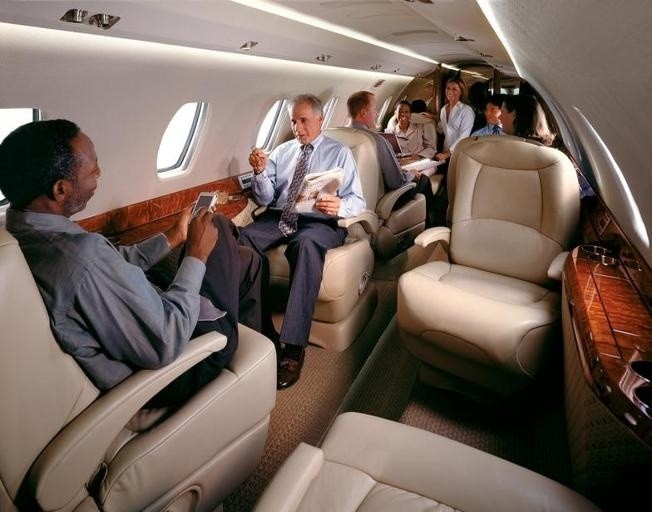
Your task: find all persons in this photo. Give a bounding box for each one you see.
[232,92,368,391]
[1,117,268,407]
[463,80,493,132]
[408,98,428,114]
[468,93,507,138]
[345,90,439,229]
[388,100,438,178]
[417,75,477,228]
[496,93,556,150]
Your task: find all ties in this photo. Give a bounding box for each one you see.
[278,146,310,237]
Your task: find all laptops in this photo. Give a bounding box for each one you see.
[378,132,412,158]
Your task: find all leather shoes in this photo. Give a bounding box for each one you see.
[276,346,306,390]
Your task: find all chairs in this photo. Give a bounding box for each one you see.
[444,133,550,225]
[1,227,280,512]
[366,133,427,266]
[250,411,603,512]
[261,129,382,351]
[397,136,580,397]
[387,109,438,159]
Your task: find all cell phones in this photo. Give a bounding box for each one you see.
[188,192,218,225]
